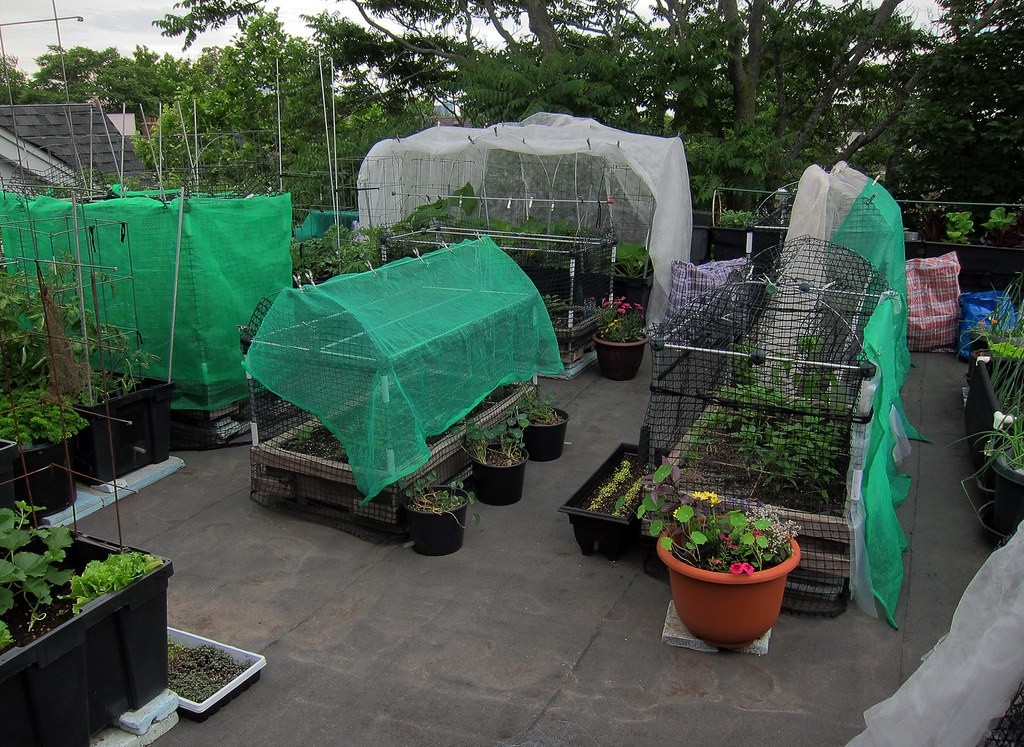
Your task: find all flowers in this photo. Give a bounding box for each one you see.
[585,295,647,342]
[609,462,802,575]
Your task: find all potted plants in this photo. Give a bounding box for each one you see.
[0,249,176,747]
[556,442,671,557]
[443,410,528,504]
[512,381,569,463]
[944,268,1024,550]
[711,207,790,262]
[395,467,472,556]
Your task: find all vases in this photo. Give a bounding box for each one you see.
[656,517,802,648]
[593,331,651,380]
[690,226,712,266]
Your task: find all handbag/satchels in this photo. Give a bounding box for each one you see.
[900,251,960,352]
[663,259,745,337]
[957,290,1016,358]
